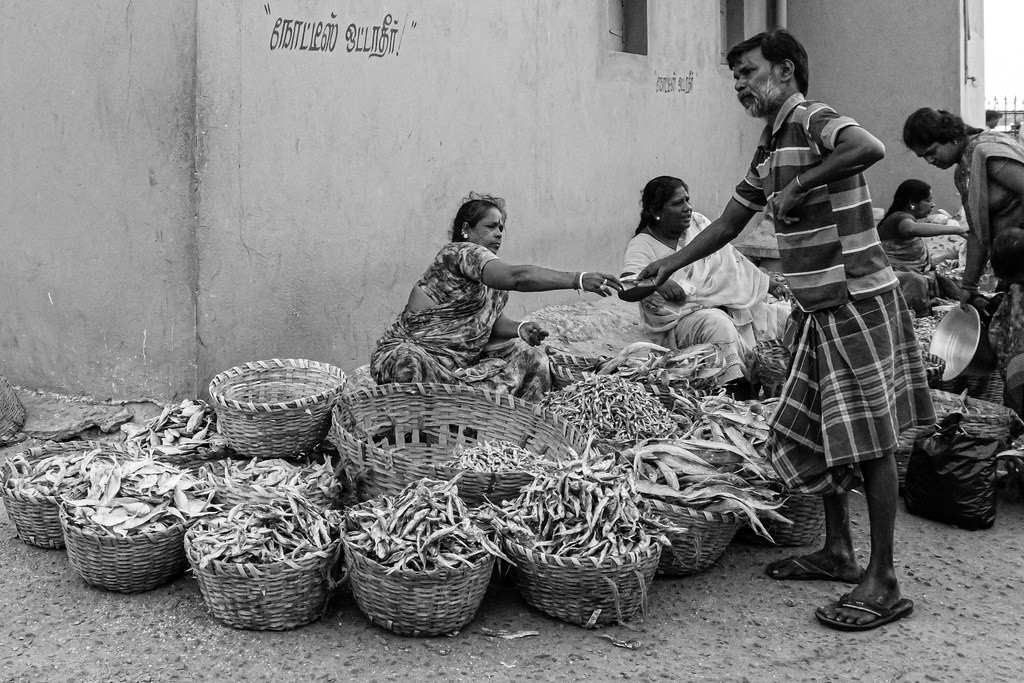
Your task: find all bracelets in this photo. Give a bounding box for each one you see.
[517,320,530,338]
[574,271,587,295]
[795,172,806,190]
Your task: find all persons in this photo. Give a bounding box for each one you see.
[369,192,627,442]
[877,107,1024,488]
[631,29,942,632]
[617,176,790,400]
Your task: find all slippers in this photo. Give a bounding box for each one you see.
[766,553,866,583]
[815,592,916,631]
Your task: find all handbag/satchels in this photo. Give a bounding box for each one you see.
[903,414,999,530]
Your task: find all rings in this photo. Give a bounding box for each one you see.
[603,278,608,285]
[600,284,607,291]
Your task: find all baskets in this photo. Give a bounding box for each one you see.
[0,356,829,636]
[887,259,1018,494]
[752,339,791,398]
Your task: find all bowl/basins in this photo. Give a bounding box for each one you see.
[929,304,996,383]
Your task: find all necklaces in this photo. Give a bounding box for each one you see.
[647,223,676,250]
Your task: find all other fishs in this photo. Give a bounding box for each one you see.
[440,438,557,475]
[4,395,230,537]
[343,467,518,575]
[533,342,794,544]
[502,430,687,556]
[186,456,349,568]
